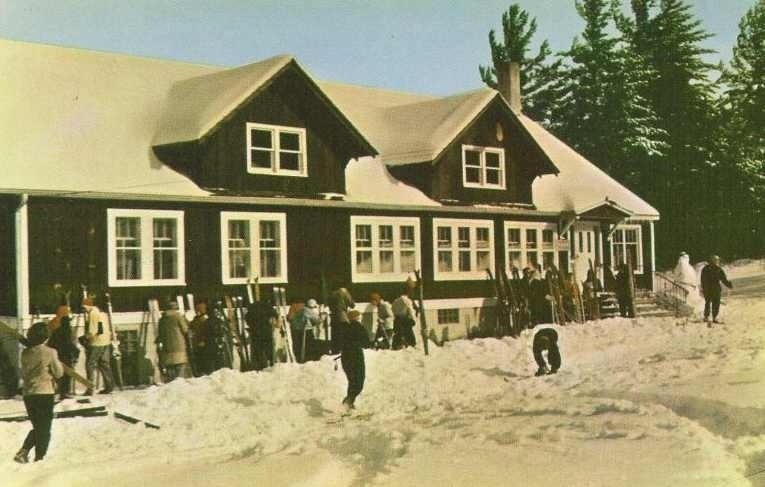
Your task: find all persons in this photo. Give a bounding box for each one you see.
[339,306,378,409]
[533,329,561,376]
[371,285,416,349]
[48,299,113,398]
[613,264,634,319]
[287,277,354,365]
[154,297,279,382]
[520,268,544,328]
[11,322,64,464]
[699,251,734,324]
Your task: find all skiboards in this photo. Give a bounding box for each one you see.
[484,257,603,337]
[147,277,296,385]
[316,271,332,359]
[414,269,430,357]
[0,284,124,422]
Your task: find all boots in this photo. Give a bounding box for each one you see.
[15,448,28,462]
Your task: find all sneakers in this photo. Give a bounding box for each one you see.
[343,397,353,411]
[63,388,112,397]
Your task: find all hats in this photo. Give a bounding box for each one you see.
[370,292,379,302]
[82,299,92,305]
[307,299,316,307]
[348,310,360,319]
[28,323,48,345]
[196,304,206,313]
